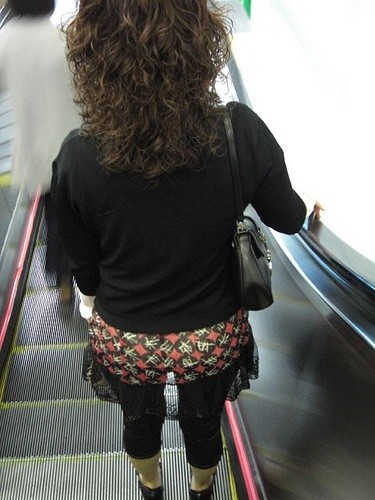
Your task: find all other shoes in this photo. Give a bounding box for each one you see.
[133,462,163,500]
[187,464,217,500]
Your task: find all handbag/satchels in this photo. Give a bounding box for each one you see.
[218,106,277,313]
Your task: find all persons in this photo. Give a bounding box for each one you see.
[0,0,85,304]
[51,0,326,499]
